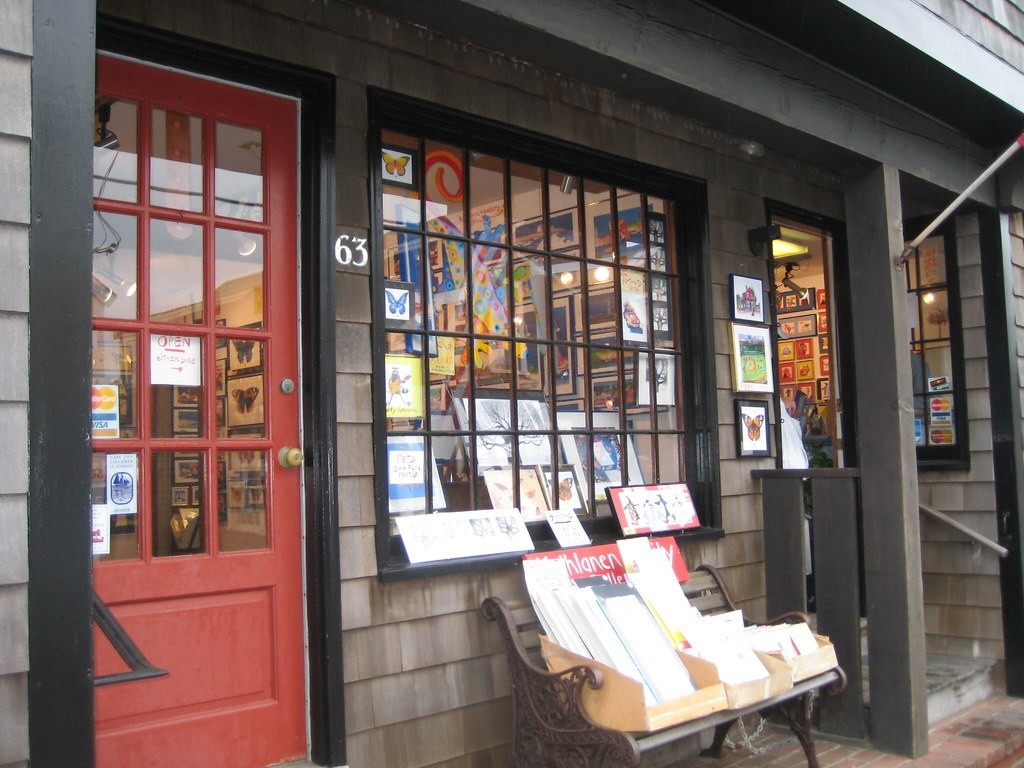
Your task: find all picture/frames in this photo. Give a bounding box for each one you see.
[728,274,766,325]
[780,384,797,400]
[386,435,451,517]
[536,463,590,517]
[776,287,816,315]
[795,338,814,360]
[778,341,796,362]
[779,362,795,383]
[380,141,419,192]
[727,321,775,394]
[777,313,817,340]
[797,382,816,402]
[479,465,551,526]
[796,360,815,381]
[815,289,829,354]
[91,318,266,509]
[604,481,703,539]
[386,193,677,481]
[816,355,830,407]
[556,409,645,504]
[732,399,772,459]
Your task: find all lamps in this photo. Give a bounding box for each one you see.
[94,135,120,157]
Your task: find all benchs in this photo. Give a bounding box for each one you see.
[480,564,847,768]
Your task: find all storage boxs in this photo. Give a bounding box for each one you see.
[719,649,792,710]
[769,633,838,682]
[537,631,729,734]
